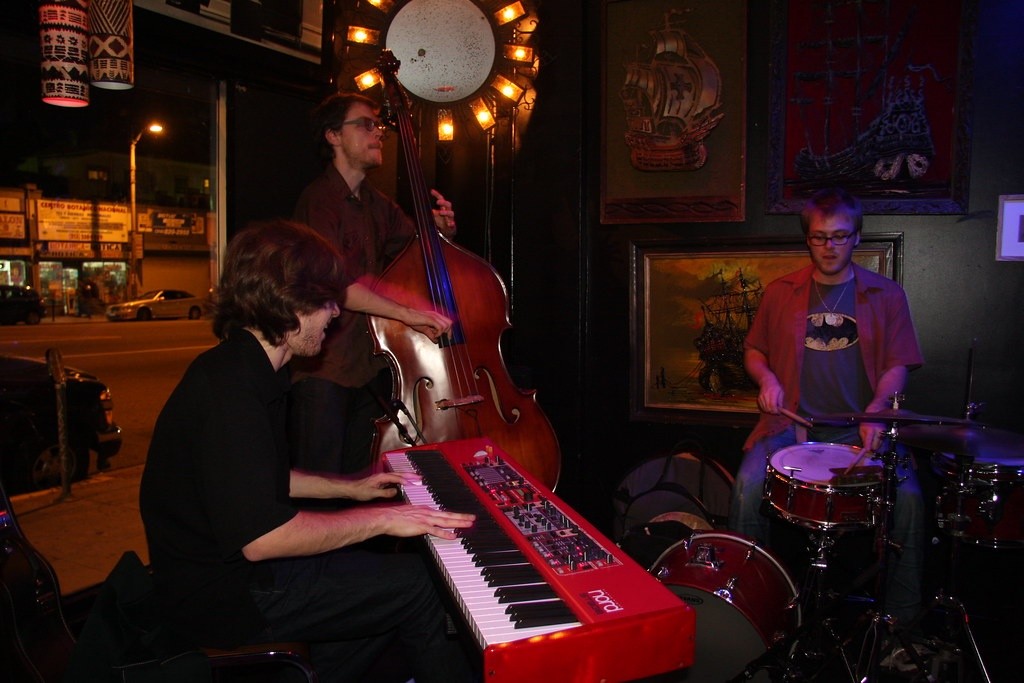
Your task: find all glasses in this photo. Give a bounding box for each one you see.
[805,226,865,247]
[343,117,388,132]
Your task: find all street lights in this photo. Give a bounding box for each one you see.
[128,123,165,298]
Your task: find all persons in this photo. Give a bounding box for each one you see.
[727,188,930,670]
[292,95,457,510]
[74,278,99,318]
[138,223,477,683]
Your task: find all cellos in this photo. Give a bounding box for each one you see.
[364,48,563,495]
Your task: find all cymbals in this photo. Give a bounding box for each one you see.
[896,423,1024,468]
[808,408,968,423]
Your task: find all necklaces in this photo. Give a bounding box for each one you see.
[814,270,851,325]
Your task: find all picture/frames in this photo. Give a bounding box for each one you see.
[623,232,905,429]
[993,193,1024,261]
[599,1,749,225]
[768,0,977,215]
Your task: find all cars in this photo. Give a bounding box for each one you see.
[104,288,208,322]
[0,285,47,327]
[0,354,123,497]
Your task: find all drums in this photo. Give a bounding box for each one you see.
[766,443,895,529]
[648,534,801,683]
[938,464,1024,550]
[609,449,738,570]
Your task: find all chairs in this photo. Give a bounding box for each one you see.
[1,493,307,683]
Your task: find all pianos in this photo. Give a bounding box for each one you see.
[379,437,697,683]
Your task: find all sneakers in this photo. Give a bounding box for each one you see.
[878,640,918,672]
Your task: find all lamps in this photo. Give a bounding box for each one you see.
[89,0,136,92]
[38,0,90,107]
[341,1,539,144]
